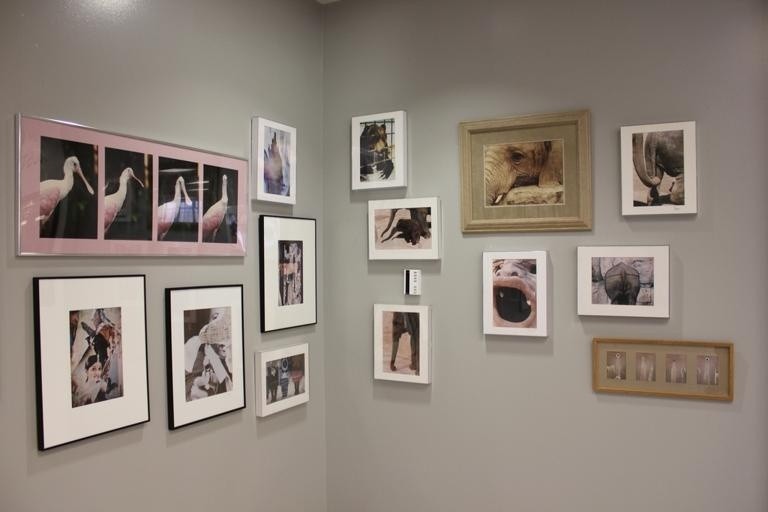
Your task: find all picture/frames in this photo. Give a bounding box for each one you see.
[14,112,251,258]
[350,111,407,192]
[455,108,598,238]
[366,199,441,262]
[259,214,318,334]
[253,342,311,420]
[617,120,701,221]
[33,273,151,451]
[251,119,298,207]
[480,249,549,339]
[585,336,733,401]
[163,283,246,430]
[372,303,431,386]
[577,244,673,322]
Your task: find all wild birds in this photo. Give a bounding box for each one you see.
[40,156,95,227]
[203,174,229,242]
[104,167,145,233]
[157,176,193,240]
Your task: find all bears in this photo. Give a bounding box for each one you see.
[360,124,394,182]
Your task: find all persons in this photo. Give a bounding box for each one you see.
[74,355,107,405]
[266,359,302,402]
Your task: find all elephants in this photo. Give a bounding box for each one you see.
[483,140,563,206]
[381,207,431,239]
[381,219,421,246]
[632,129,684,206]
[390,312,419,375]
